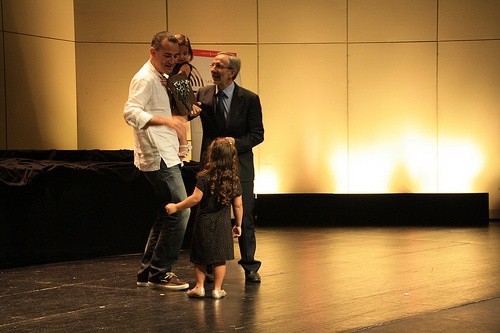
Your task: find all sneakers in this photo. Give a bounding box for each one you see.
[136,263,150,286]
[148,265,189,291]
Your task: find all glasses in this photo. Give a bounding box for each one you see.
[209,64,235,71]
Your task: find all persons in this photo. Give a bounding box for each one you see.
[165,138,243,299]
[123,31,193,290]
[188,51,264,281]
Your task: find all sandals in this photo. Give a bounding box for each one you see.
[178,143,191,159]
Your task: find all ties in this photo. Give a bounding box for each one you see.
[215,90,226,137]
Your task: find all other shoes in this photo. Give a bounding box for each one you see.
[211,290,227,299]
[186,287,205,298]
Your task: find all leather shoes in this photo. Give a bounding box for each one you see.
[245,271,261,282]
[206,273,214,283]
